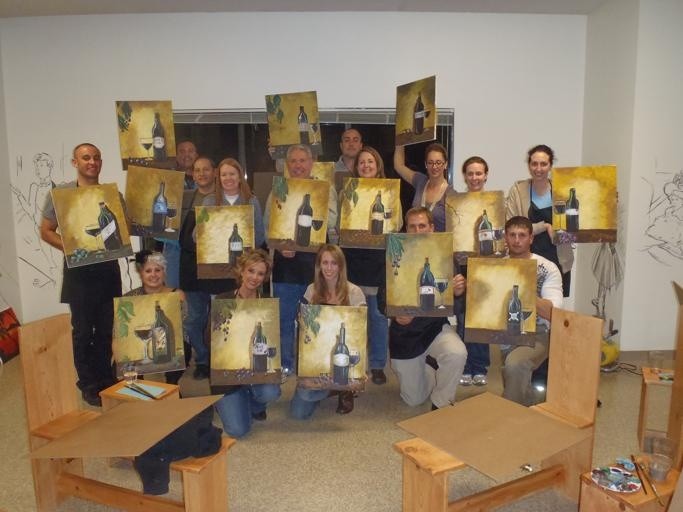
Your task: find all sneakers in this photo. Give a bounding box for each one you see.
[79,378,119,407]
[327,388,356,415]
[252,410,267,422]
[370,368,389,385]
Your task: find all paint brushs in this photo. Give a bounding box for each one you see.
[631,454,665,506]
[124,382,158,400]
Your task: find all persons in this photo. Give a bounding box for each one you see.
[39,129,575,438]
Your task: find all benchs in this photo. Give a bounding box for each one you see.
[30,408,235,512]
[392,401,596,512]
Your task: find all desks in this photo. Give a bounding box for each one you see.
[637,367,674,452]
[577,451,680,512]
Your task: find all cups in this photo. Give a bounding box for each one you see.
[647,455,673,481]
[648,350,665,374]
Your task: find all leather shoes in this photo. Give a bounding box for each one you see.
[457,373,488,388]
